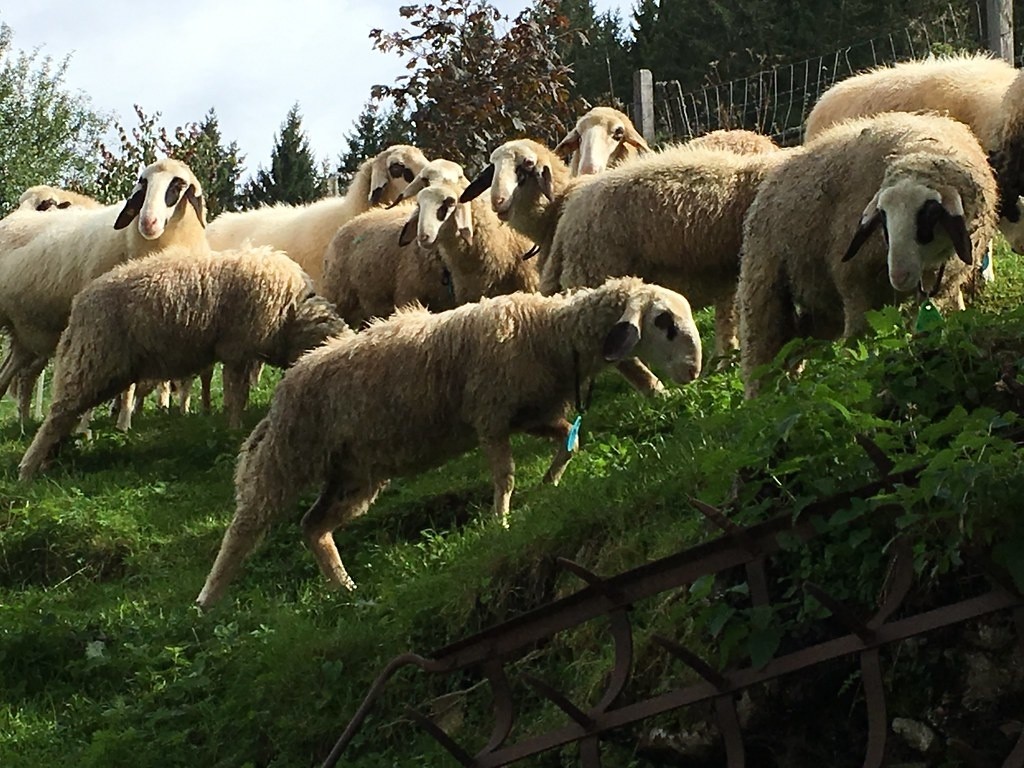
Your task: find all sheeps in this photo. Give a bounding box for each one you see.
[0,47,1024,615]
